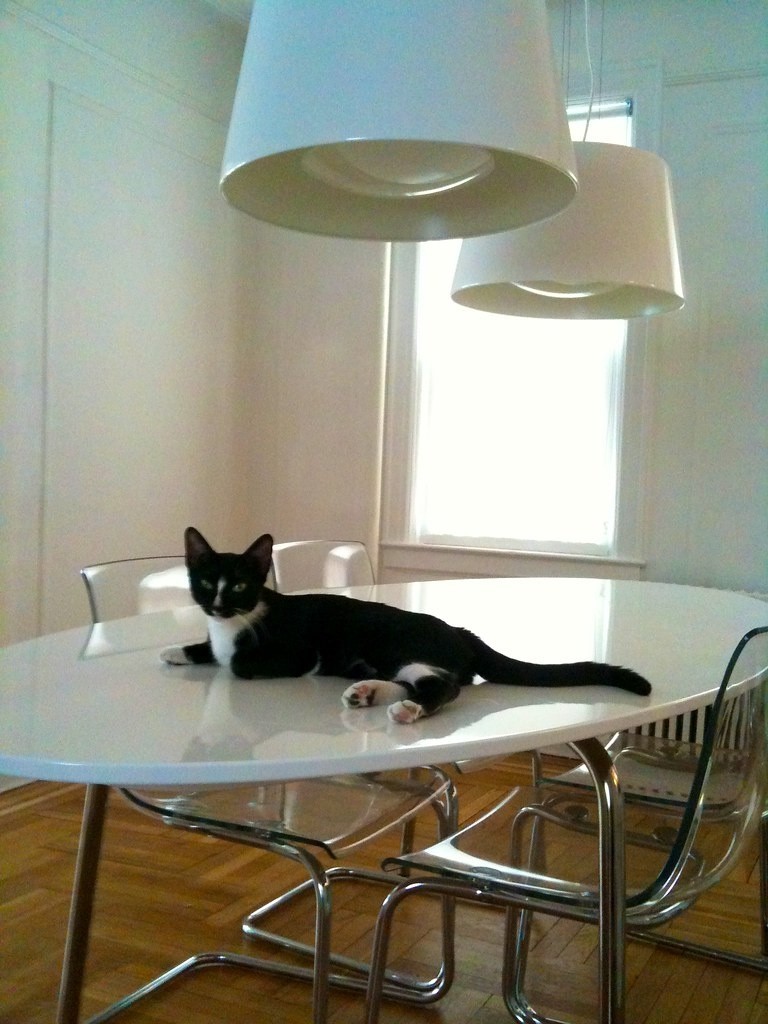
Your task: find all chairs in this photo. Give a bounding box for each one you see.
[81,537,768,1024]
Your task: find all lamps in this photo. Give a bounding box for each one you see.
[217,0,582,242]
[447,0,689,321]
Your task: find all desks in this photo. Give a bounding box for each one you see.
[0,575,768,1023]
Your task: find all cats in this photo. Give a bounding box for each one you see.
[158,525,652,726]
